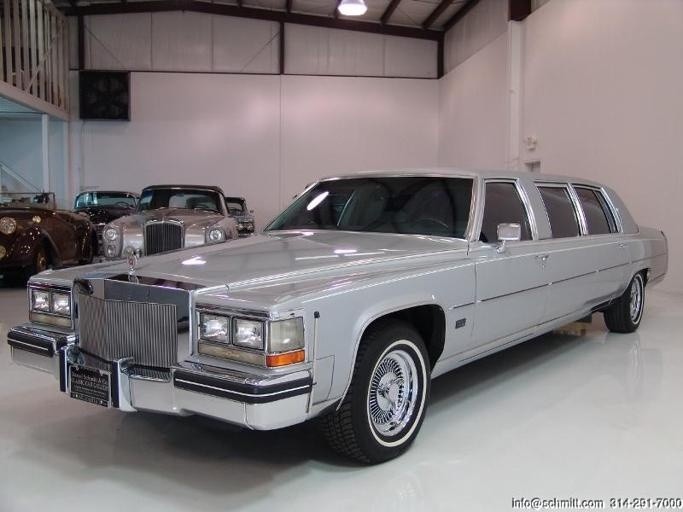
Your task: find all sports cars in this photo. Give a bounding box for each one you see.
[0,191,99,284]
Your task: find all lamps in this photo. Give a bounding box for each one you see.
[337,0,368,16]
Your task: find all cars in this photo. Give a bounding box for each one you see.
[72,183,256,262]
[5,165,669,469]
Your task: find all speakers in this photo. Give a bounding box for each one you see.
[77,69,133,122]
